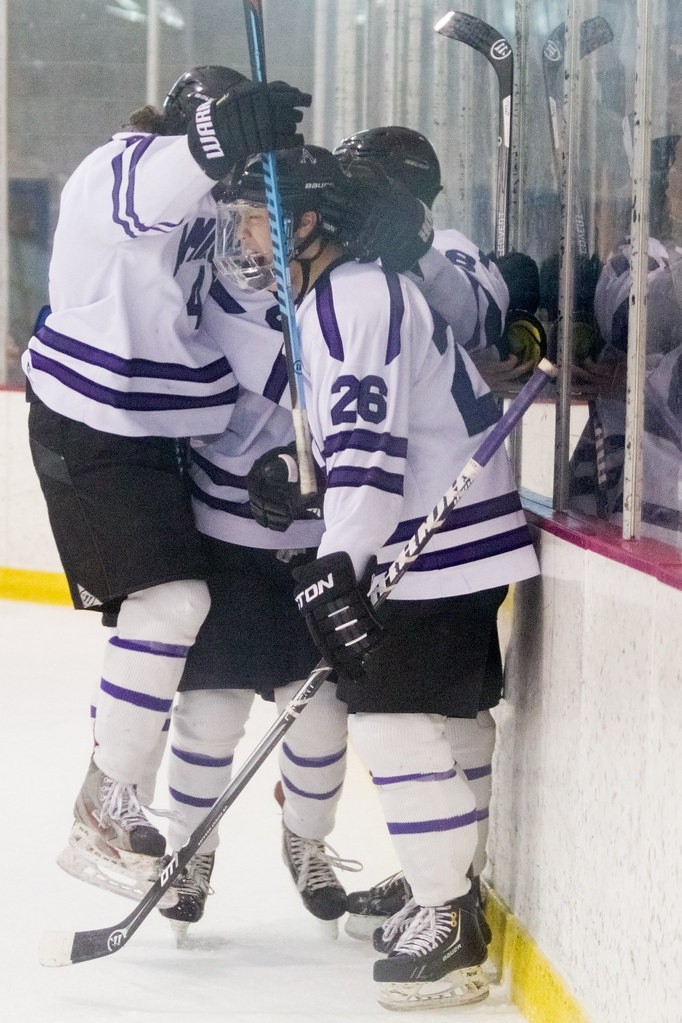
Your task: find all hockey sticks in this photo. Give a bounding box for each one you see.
[433,8,518,254]
[243,1,320,500]
[542,14,615,495]
[36,357,558,967]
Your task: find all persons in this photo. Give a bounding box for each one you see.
[544,136,682,549]
[22,66,549,1010]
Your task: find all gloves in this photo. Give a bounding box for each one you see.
[189,78,311,181]
[247,439,328,532]
[314,149,435,273]
[294,552,402,684]
[540,251,601,318]
[496,247,539,314]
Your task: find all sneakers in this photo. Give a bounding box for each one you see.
[373,894,498,981]
[373,876,492,1009]
[275,781,364,941]
[55,759,179,909]
[157,852,215,949]
[344,868,484,943]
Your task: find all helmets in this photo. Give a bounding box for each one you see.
[334,125,440,209]
[162,65,250,132]
[211,144,334,294]
